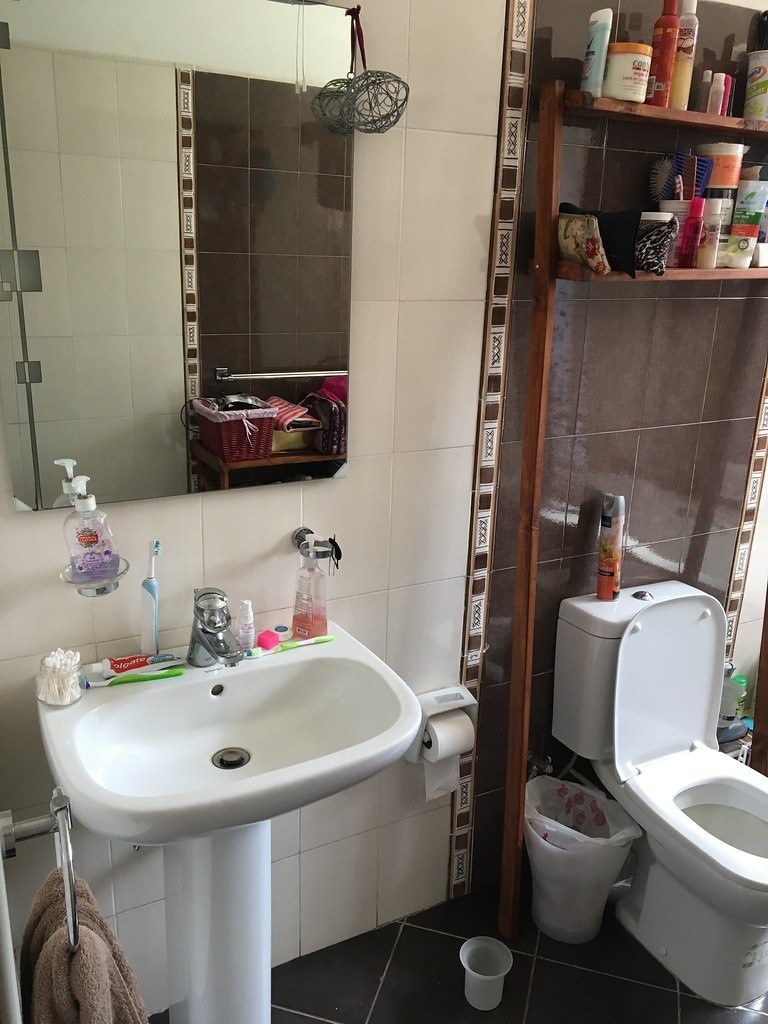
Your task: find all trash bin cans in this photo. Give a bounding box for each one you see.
[521,774,642,944]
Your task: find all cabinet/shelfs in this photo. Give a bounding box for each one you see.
[498,81,768,937]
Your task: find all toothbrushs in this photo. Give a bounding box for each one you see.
[140,539,166,657]
[63,669,187,691]
[673,175,685,202]
[245,634,337,660]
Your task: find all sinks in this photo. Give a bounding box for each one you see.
[33,608,423,849]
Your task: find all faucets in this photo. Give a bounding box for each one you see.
[184,584,244,672]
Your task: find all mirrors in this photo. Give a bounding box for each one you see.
[1,0,355,512]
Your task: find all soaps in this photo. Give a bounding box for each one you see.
[256,626,280,650]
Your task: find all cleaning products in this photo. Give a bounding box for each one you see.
[594,491,626,601]
[730,672,748,725]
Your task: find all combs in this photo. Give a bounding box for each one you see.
[681,155,697,200]
[675,151,714,202]
[649,154,676,202]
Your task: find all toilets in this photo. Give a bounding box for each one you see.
[550,578,768,1011]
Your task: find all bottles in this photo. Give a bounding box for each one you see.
[35,655,87,708]
[732,675,747,695]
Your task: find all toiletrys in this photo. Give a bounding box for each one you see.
[82,652,187,679]
[63,474,124,599]
[578,0,768,127]
[52,456,78,509]
[637,175,767,269]
[291,532,332,643]
[234,597,258,652]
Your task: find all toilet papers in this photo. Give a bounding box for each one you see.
[422,710,478,804]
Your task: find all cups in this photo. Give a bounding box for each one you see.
[743,50,768,121]
[459,937,513,1011]
[659,197,693,268]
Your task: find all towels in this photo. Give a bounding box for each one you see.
[15,866,151,1024]
